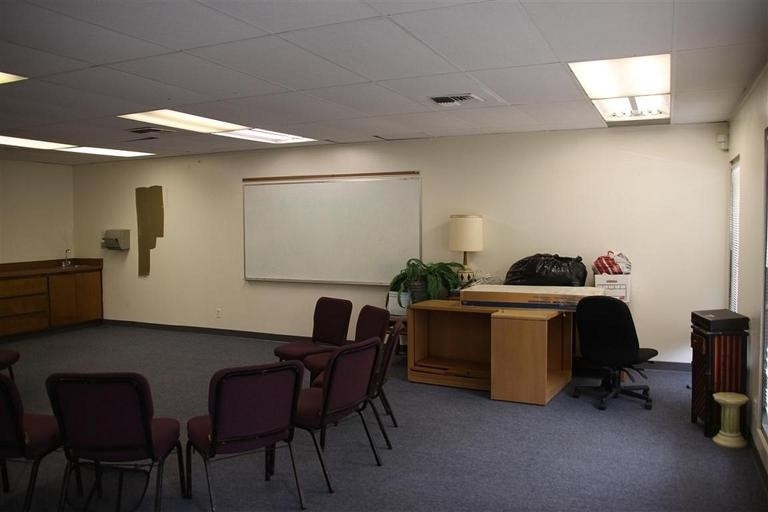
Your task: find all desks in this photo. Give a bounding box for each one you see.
[406,298,573,406]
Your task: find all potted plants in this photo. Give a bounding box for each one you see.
[385,257,466,315]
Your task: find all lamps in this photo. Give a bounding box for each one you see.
[447,215,483,285]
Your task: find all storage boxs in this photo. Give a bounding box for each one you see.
[595,273,630,303]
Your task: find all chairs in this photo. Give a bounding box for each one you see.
[570,295,659,411]
[185,359,305,511]
[46,372,186,511]
[273,296,353,363]
[270,336,383,494]
[1,346,65,511]
[311,319,404,427]
[302,305,390,388]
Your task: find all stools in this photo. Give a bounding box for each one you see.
[379,316,407,383]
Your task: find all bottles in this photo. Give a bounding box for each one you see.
[65,249,71,268]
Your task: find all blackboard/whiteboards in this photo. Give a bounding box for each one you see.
[241,176,423,286]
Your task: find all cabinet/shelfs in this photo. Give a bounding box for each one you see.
[689,308,751,439]
[0,275,49,336]
[48,269,102,329]
